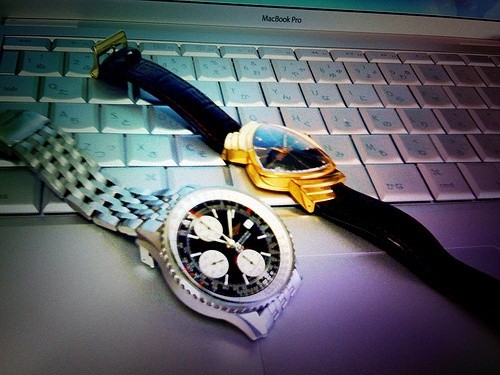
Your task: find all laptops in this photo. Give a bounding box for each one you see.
[0,0,500,375]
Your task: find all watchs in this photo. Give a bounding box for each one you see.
[89,30,500,344]
[0,106,304,341]
